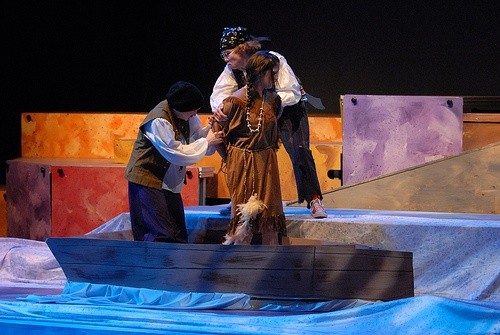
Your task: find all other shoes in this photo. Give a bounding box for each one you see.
[308,200,328,219]
[220,202,231,215]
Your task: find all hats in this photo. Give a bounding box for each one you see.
[167,81,204,112]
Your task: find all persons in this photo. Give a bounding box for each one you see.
[205,27,328,218]
[124,82,224,243]
[207,50,287,245]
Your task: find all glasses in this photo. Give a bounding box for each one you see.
[219,46,238,61]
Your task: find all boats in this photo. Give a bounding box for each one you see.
[45,226,414,304]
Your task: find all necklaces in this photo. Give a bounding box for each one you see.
[247,84,264,133]
